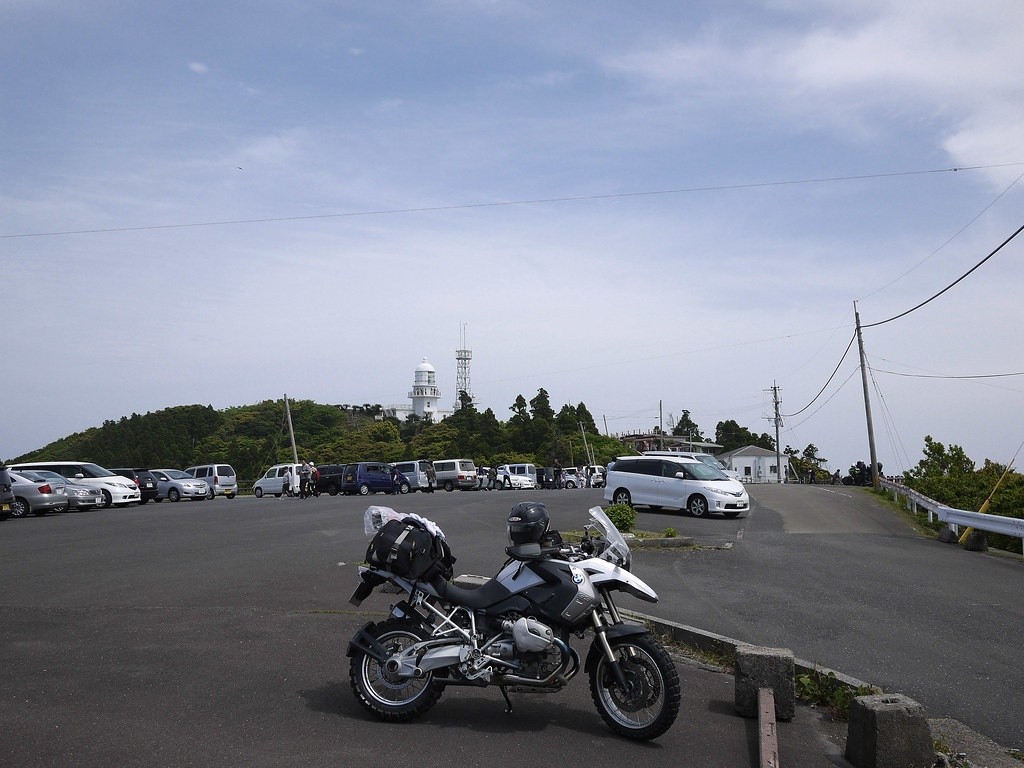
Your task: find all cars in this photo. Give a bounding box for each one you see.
[0,460,15,521]
[5,470,68,519]
[14,469,104,513]
[342,459,608,496]
[149,468,209,502]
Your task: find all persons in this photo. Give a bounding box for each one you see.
[485,464,495,491]
[607,455,616,470]
[280,467,295,501]
[298,459,310,499]
[425,462,437,493]
[553,459,562,489]
[585,464,592,488]
[831,469,840,485]
[492,465,498,490]
[502,462,513,490]
[809,466,817,485]
[576,465,585,488]
[307,461,320,498]
[390,465,401,495]
[478,463,483,490]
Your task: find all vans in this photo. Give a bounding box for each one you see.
[642,448,739,482]
[6,461,142,509]
[602,455,750,518]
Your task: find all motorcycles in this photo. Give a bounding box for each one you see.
[345,505,683,741]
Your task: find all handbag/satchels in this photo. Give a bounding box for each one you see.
[365,516,457,582]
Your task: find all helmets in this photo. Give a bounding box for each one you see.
[507,500,551,545]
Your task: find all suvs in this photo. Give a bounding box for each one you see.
[184,464,239,501]
[252,464,316,497]
[314,464,353,496]
[108,468,160,506]
